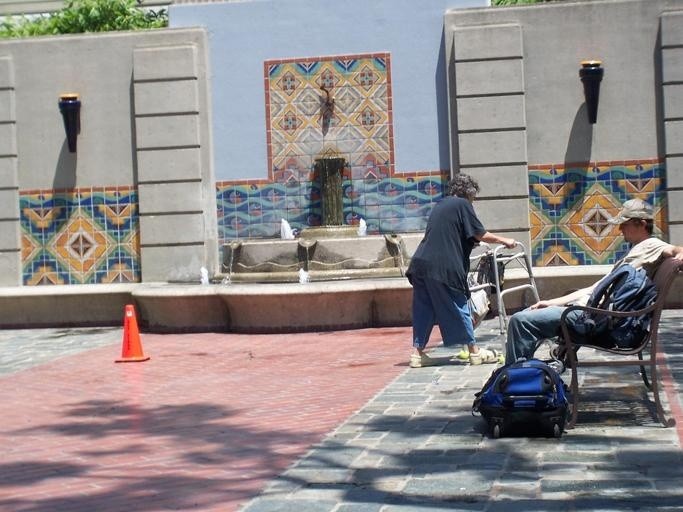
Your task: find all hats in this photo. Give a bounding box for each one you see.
[606,197,655,225]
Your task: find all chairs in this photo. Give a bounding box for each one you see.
[561,253,683,448]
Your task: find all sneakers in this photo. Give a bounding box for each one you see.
[468,348,498,366]
[409,353,438,368]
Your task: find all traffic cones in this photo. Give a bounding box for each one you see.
[115,305,149,362]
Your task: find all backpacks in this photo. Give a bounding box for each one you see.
[555,264,659,389]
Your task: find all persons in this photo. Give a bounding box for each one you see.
[505,198,683,368]
[405,173,517,368]
